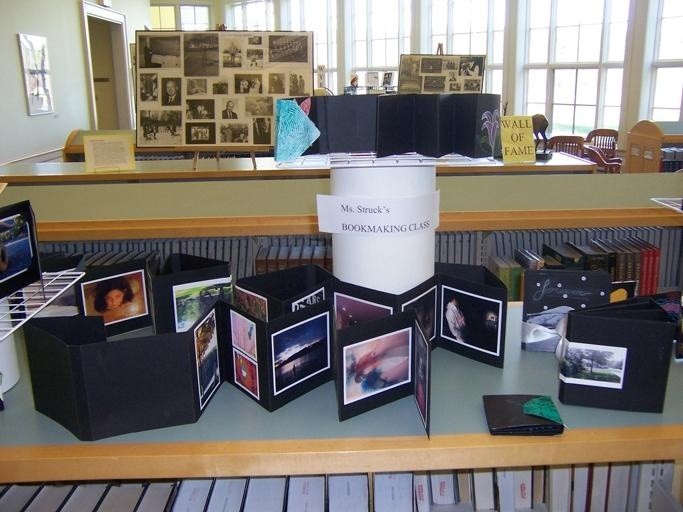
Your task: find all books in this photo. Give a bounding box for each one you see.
[33,233,332,289]
[2,459,674,511]
[432,224,682,303]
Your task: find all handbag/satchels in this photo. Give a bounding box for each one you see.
[483,395,562,435]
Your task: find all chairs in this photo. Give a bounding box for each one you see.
[548,128,622,173]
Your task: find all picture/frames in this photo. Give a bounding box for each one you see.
[82,133,136,172]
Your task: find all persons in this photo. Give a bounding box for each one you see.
[450,72,457,81]
[460,59,479,77]
[296,74,306,94]
[222,100,238,118]
[227,41,238,65]
[188,79,205,96]
[164,81,180,105]
[194,105,208,119]
[200,104,206,112]
[94,277,134,324]
